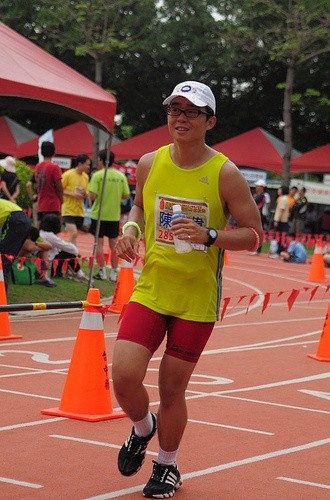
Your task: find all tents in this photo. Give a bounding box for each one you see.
[0,115,330,182]
[0,21,116,294]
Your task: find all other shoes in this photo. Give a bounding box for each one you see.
[73,275,89,283]
[108,276,116,282]
[270,253,279,258]
[249,251,260,256]
[93,273,106,280]
[40,279,56,288]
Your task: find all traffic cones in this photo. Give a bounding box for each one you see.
[1,256,24,340]
[39,288,130,422]
[105,251,138,314]
[304,301,330,364]
[304,237,330,284]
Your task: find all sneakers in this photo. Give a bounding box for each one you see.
[142,460,183,498]
[118,412,158,477]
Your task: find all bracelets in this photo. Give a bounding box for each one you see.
[122,221,142,241]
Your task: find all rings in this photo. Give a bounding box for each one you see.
[186,236,190,241]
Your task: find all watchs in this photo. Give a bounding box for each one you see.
[203,227,218,248]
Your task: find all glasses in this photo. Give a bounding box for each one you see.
[167,107,211,118]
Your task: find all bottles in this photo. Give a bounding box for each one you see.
[171,204,191,254]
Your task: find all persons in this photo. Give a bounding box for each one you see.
[87,150,130,282]
[322,236,330,264]
[0,198,32,290]
[0,156,21,202]
[60,154,91,244]
[26,141,63,230]
[18,213,94,288]
[247,179,308,259]
[280,233,307,263]
[112,82,264,498]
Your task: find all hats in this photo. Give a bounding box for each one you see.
[0,156,16,173]
[162,81,216,116]
[254,180,265,187]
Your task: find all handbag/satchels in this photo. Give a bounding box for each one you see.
[269,239,278,253]
